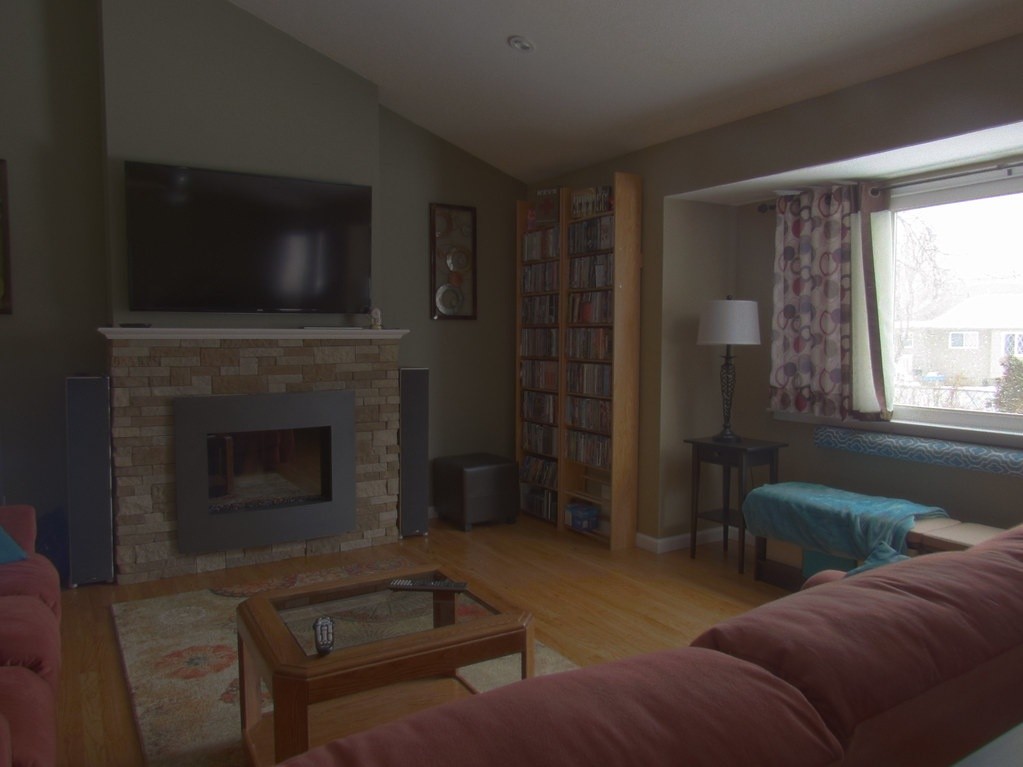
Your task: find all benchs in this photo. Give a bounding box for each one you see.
[743,479,1004,589]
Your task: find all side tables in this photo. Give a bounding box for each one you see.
[684,438,792,574]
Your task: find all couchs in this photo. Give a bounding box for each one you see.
[258,517,1023,766]
[0,506,63,766]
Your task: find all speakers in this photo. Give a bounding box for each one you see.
[396,366,431,539]
[66,374,114,583]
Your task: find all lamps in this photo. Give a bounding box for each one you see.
[696,296,761,440]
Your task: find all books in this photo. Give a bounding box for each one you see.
[521,294,558,324]
[520,227,560,262]
[568,215,615,254]
[566,361,612,397]
[569,183,611,219]
[567,291,613,323]
[564,430,611,467]
[521,261,558,291]
[521,421,558,456]
[521,359,558,389]
[526,187,559,229]
[520,328,558,356]
[577,464,610,501]
[521,391,559,425]
[563,502,600,530]
[570,256,613,289]
[518,484,557,519]
[567,327,612,362]
[520,454,558,487]
[566,397,611,433]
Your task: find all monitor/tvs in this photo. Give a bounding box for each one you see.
[123,160,373,315]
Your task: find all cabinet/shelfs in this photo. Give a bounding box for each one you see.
[518,171,645,551]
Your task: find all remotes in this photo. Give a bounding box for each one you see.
[315,615,334,654]
[388,577,468,592]
[121,322,151,328]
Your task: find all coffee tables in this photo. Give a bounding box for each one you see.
[234,559,537,764]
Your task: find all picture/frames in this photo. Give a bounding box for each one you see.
[428,202,477,320]
[0,160,12,317]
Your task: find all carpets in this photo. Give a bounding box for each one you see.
[111,556,581,765]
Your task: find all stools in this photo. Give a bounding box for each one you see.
[433,453,519,531]
